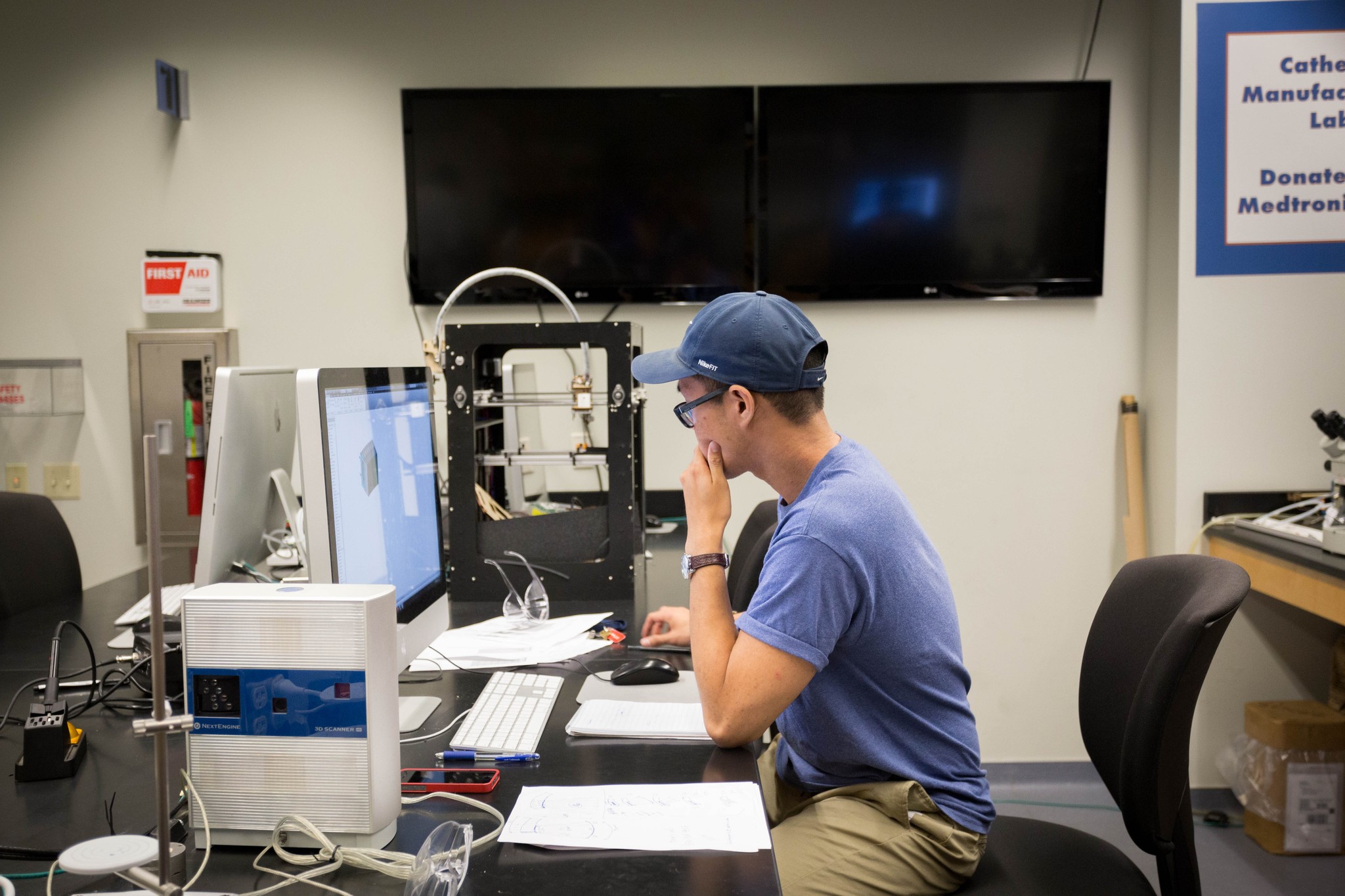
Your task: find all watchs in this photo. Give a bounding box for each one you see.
[681,552,730,580]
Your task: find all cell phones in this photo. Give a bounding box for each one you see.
[398,769,500,794]
[626,645,691,653]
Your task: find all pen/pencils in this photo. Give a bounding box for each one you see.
[434,749,540,764]
[32,676,131,692]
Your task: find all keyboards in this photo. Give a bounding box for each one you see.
[448,671,565,756]
[114,583,194,626]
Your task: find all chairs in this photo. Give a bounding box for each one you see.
[726,497,784,612]
[954,551,1253,896]
[0,489,81,621]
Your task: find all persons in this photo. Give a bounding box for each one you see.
[633,291,997,896]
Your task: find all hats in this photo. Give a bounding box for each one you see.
[630,291,828,393]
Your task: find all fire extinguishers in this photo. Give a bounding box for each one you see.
[182,380,207,516]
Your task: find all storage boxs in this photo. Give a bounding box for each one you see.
[1240,700,1344,856]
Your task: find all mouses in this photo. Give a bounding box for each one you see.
[132,613,181,633]
[611,657,679,684]
[645,514,662,528]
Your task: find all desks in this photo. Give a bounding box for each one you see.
[0,517,782,896]
[1209,497,1345,630]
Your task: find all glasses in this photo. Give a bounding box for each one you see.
[674,385,731,428]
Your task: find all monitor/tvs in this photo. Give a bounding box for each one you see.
[191,366,308,590]
[294,366,450,733]
[400,85,754,305]
[755,79,1112,298]
